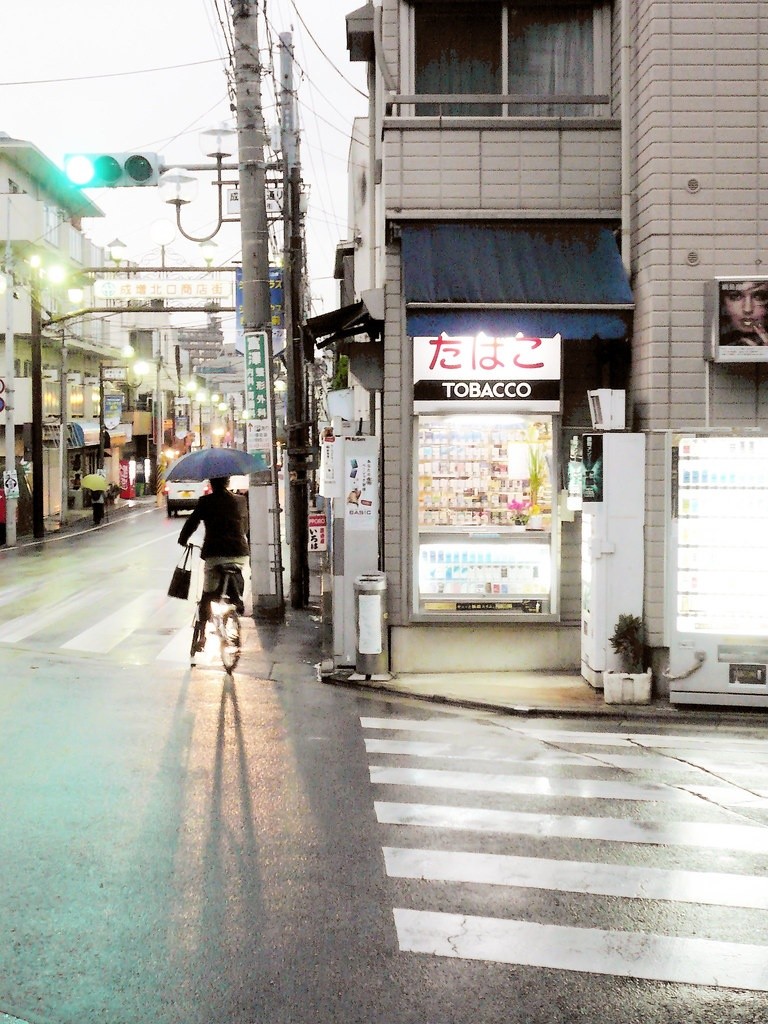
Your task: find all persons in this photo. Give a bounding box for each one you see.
[90,490,107,525]
[177,477,249,652]
[719,281,768,346]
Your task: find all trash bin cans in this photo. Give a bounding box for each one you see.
[355,570,388,675]
[136,483,144,495]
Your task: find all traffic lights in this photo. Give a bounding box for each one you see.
[64,150,159,187]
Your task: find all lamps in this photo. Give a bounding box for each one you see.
[108,239,124,268]
[163,122,291,242]
[326,357,355,422]
[199,241,219,266]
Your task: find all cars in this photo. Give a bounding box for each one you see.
[163,476,212,518]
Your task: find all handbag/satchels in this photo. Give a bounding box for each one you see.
[168,543,193,600]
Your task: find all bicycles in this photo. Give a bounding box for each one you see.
[178,541,245,673]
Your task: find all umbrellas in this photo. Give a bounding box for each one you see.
[163,442,271,484]
[81,474,110,492]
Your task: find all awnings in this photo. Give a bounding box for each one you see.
[305,300,374,351]
[398,219,637,341]
[41,420,133,451]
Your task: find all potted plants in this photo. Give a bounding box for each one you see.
[603,613,652,704]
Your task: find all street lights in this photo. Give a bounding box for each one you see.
[185,382,205,454]
